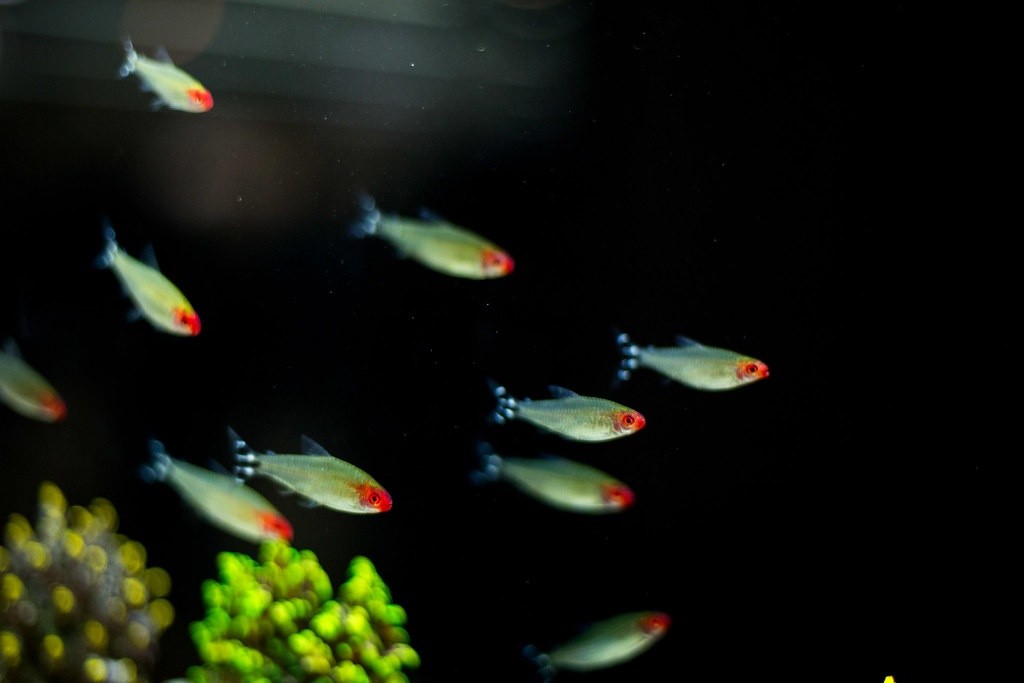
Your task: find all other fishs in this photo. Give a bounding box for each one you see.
[0,36,771,671]
